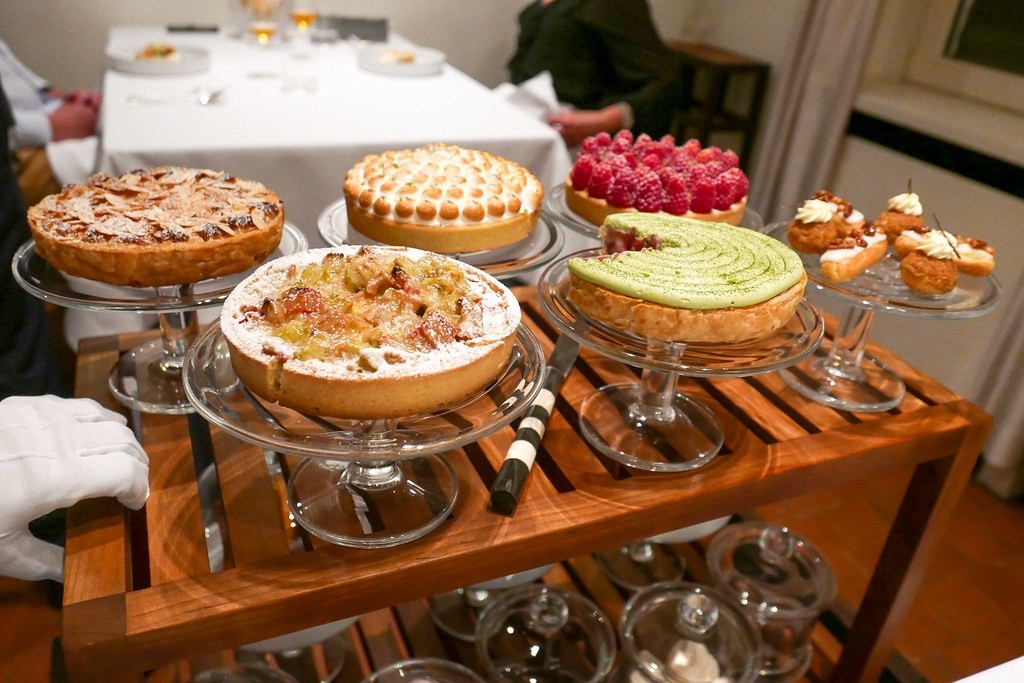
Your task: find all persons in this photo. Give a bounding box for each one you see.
[0,34,99,400]
[505,0,680,159]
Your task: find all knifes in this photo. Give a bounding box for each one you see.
[490,309,587,516]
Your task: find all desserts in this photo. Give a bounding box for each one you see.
[788,178,996,295]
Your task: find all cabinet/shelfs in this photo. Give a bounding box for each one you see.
[662,39,775,175]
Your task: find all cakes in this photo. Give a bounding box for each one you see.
[566,212,807,342]
[343,143,544,254]
[566,127,749,229]
[27,166,285,288]
[219,244,522,420]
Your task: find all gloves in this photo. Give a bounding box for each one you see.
[0,393,149,584]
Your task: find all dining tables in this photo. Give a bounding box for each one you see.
[94,26,572,258]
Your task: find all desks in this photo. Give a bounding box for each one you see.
[61,281,993,683]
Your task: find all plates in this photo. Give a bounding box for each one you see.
[358,44,446,76]
[106,41,211,74]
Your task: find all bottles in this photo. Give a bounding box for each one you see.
[191,512,835,682]
